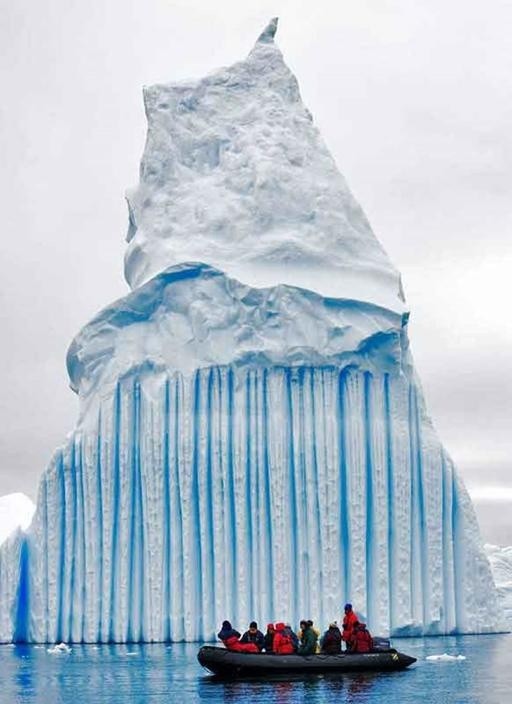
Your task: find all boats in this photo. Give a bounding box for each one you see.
[197,645,417,676]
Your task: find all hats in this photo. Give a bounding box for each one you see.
[344,603,352,609]
[300,619,313,627]
[329,621,367,630]
[266,623,291,631]
[222,620,231,627]
[250,621,257,628]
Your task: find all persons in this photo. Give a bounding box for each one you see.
[239,621,267,651]
[217,620,261,653]
[340,602,360,653]
[264,618,374,655]
